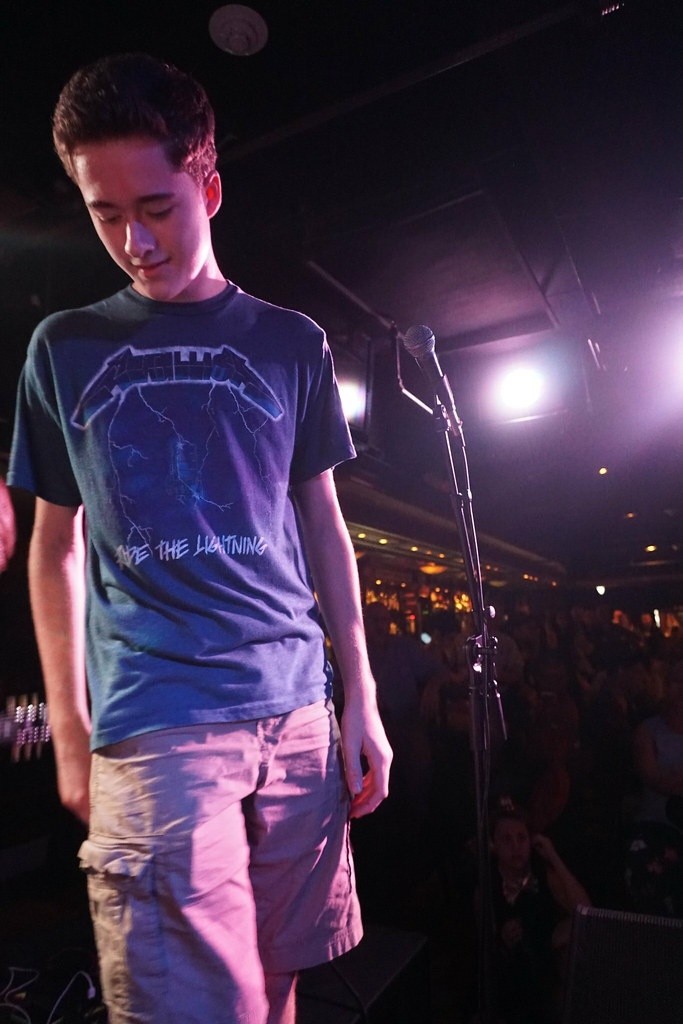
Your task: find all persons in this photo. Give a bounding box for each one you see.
[361,595,682,1024]
[4,54,394,1024]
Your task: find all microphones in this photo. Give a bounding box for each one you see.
[402,323,465,449]
